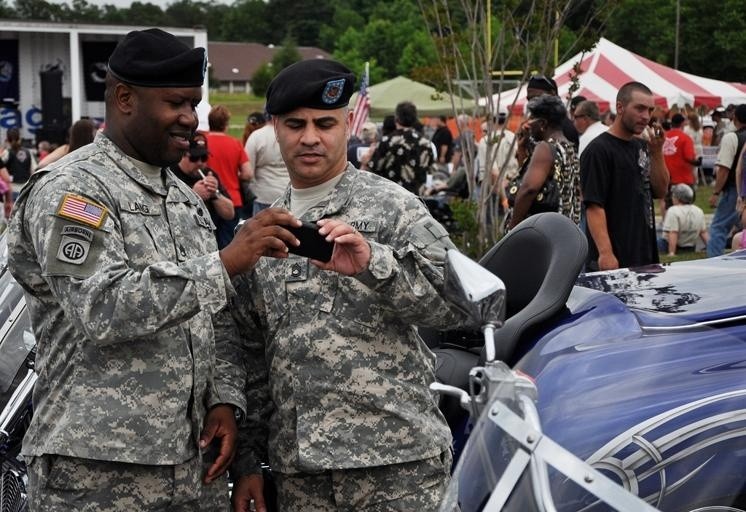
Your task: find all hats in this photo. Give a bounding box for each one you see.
[106,25,208,91]
[670,183,696,203]
[188,131,210,158]
[265,56,359,116]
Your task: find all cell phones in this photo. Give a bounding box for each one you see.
[276,217,336,264]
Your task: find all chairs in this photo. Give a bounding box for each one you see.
[406,209,592,433]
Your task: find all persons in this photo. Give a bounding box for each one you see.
[6,28,245,510]
[223,60,481,510]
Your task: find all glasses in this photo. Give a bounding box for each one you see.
[183,152,210,164]
[573,112,586,120]
[528,73,557,86]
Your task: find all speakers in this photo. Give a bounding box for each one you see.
[36,69,71,148]
